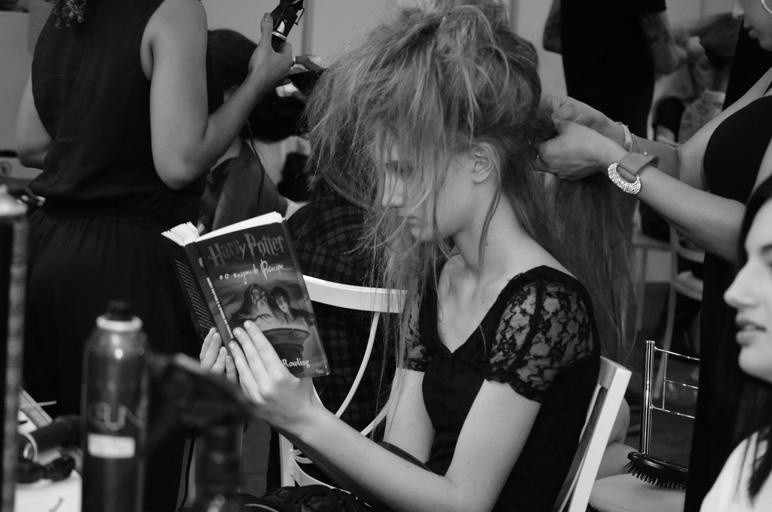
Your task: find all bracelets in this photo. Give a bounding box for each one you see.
[618,120,633,151]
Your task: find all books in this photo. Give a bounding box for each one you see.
[161,213,331,380]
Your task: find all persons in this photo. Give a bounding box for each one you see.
[653,34,711,145]
[698,176,772,512]
[677,14,743,143]
[543,0,679,140]
[640,43,712,242]
[534,0,772,512]
[14,0,294,415]
[198,1,602,512]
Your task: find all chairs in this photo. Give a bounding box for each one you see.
[278,275,408,507]
[653,224,705,400]
[550,358,631,512]
[589,340,700,512]
[621,230,670,333]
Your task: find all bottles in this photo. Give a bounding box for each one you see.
[78,301,147,512]
